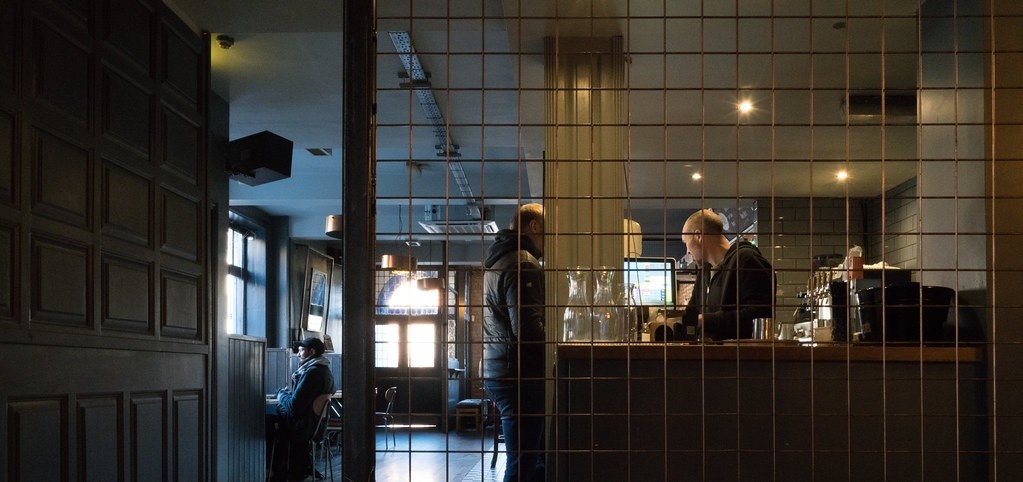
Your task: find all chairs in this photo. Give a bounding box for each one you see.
[266,386,397,482]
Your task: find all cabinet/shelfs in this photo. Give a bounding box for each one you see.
[0,2,214,482]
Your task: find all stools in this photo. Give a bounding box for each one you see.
[455,399,483,434]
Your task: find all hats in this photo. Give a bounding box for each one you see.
[293,337,325,354]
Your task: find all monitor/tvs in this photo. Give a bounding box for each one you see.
[624,257,677,310]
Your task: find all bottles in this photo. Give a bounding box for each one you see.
[566,273,571,298]
[592,266,615,341]
[562,265,592,342]
[615,283,637,342]
[640,322,651,342]
[656,313,664,322]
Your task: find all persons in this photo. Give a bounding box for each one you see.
[682,209,777,340]
[477,202,546,482]
[275,337,335,482]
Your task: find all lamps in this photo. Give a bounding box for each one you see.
[325,215,342,238]
[381,206,417,271]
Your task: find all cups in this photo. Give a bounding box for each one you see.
[778,323,794,340]
[752,318,772,340]
[806,264,844,297]
